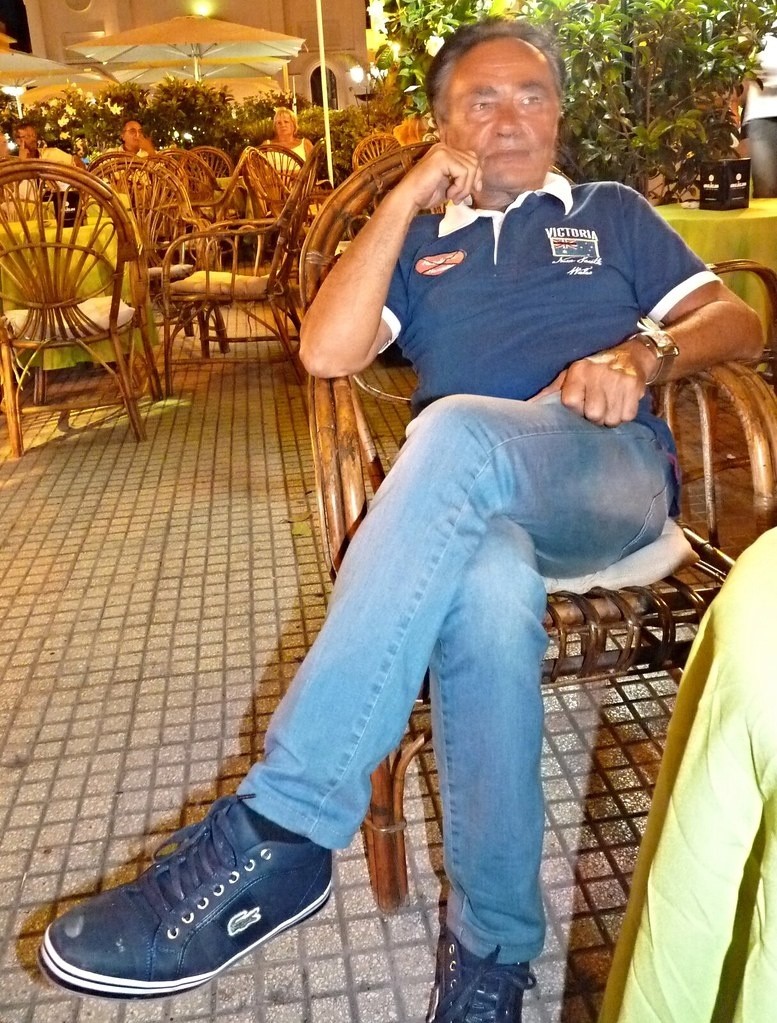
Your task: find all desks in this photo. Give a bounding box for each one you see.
[652,198,776,371]
[199,175,246,218]
[1,218,161,370]
[3,193,130,226]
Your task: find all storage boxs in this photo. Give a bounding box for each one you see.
[698,156,750,210]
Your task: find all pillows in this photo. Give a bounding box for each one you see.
[169,268,283,295]
[540,518,701,596]
[2,297,135,339]
[148,263,193,277]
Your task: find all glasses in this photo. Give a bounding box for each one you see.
[18,133,38,140]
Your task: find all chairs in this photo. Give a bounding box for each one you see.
[0,134,777,910]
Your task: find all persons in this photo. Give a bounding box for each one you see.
[100,120,155,186]
[0,120,86,202]
[243,108,314,243]
[36,18,764,1023]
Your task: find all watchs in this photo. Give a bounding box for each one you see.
[628,331,678,386]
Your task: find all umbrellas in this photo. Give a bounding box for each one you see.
[67,15,306,87]
[0,48,106,120]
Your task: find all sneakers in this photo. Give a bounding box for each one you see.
[37,792,332,998]
[425,930,536,1023]
